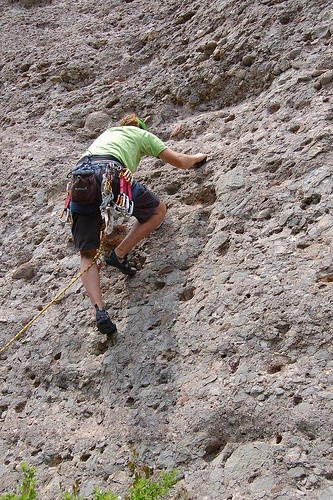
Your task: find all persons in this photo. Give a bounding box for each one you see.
[60,114,206,334]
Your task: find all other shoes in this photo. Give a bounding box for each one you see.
[104,249,137,275]
[94,304,116,334]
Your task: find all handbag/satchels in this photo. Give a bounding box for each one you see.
[71,170,98,205]
